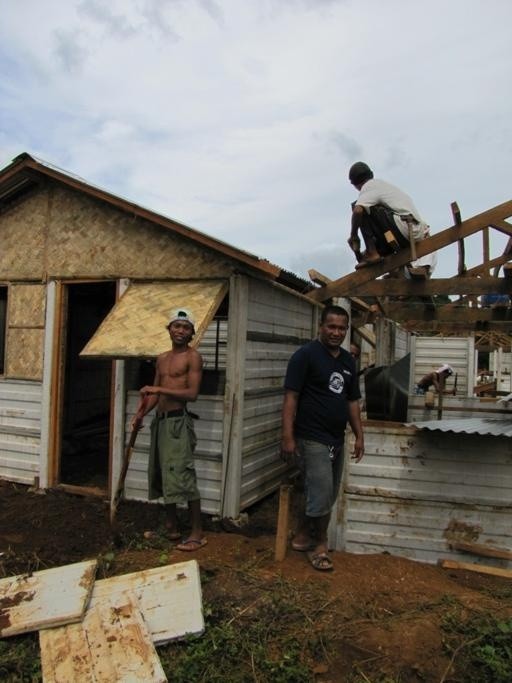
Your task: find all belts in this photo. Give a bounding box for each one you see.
[155,408,201,420]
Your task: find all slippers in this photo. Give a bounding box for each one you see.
[354,256,386,270]
[143,529,181,541]
[176,535,208,551]
[306,546,334,570]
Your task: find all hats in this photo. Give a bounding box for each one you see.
[166,308,197,336]
[348,161,372,186]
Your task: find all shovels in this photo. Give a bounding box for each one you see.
[112,393,150,547]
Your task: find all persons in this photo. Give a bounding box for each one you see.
[348,161,427,269]
[350,344,360,361]
[129,308,202,549]
[279,305,365,567]
[414,365,456,393]
